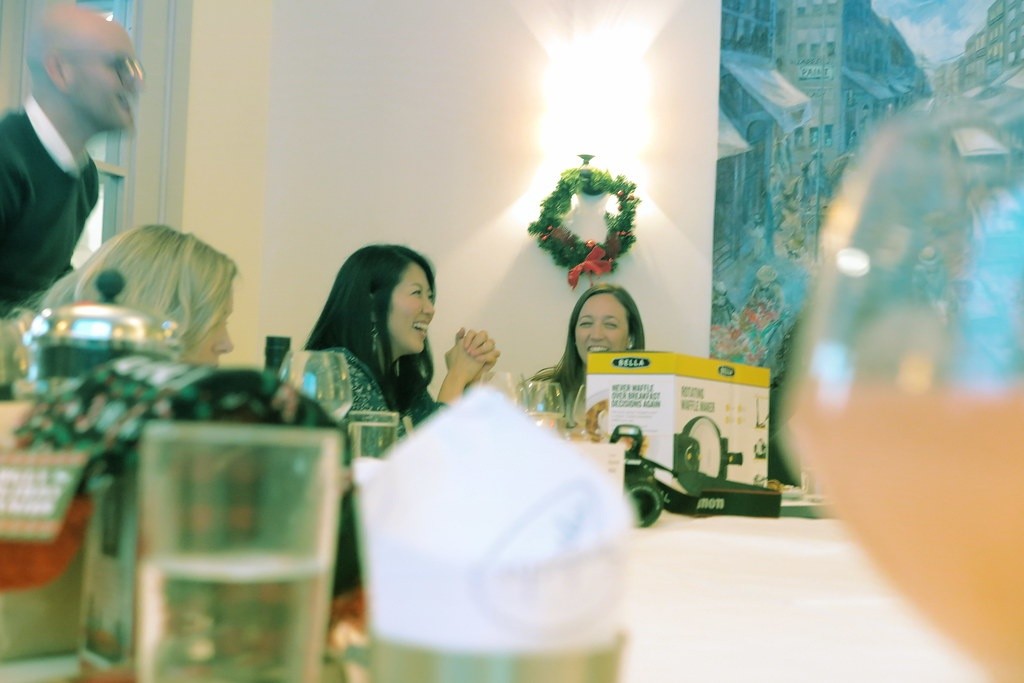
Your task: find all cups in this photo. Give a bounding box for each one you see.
[348,410,398,467]
[132,417,345,683]
[525,381,565,430]
[480,371,523,415]
[277,350,354,421]
[774,95,1024,683]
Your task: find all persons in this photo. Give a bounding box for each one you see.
[9,223,237,367]
[0,6,144,401]
[302,244,500,593]
[530,281,645,427]
[768,316,801,488]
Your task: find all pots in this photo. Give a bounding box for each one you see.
[13,273,182,393]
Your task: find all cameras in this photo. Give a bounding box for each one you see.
[609,424,663,528]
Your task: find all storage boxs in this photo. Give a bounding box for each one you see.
[586,351,771,487]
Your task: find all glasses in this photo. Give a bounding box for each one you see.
[64,49,143,86]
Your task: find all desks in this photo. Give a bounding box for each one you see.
[0,528,974,683]
